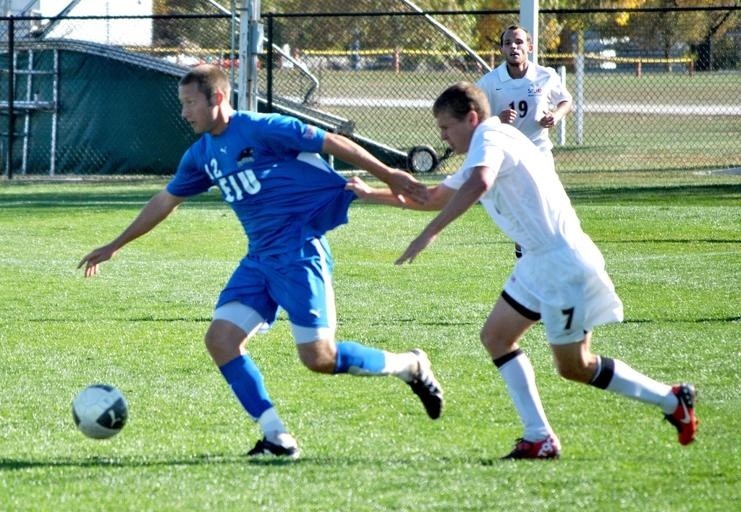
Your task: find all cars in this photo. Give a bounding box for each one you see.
[164,54,395,68]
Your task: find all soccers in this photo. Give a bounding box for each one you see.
[72,384,128,439]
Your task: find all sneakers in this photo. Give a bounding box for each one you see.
[406,347,443,420]
[244,436,301,460]
[660,383,699,446]
[498,433,562,460]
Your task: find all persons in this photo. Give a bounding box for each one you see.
[476,26,572,259]
[344,82,699,466]
[77,64,443,460]
